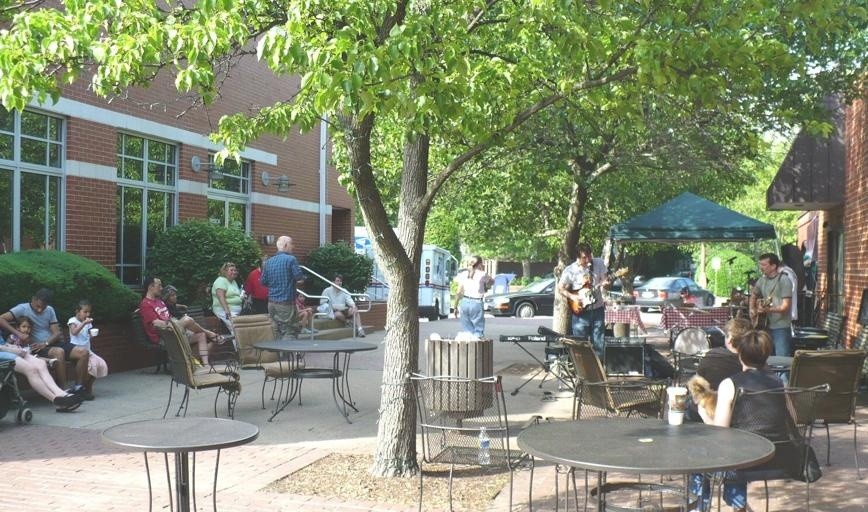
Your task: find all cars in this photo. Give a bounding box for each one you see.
[632,277,715,313]
[482,278,554,318]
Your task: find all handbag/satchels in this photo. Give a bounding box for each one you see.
[783,409,822,483]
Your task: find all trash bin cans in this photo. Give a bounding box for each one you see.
[425,336,495,429]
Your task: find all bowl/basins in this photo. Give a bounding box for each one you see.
[89,329,99,337]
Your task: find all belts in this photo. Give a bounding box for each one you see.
[463,295,483,301]
[586,306,603,313]
[268,299,297,305]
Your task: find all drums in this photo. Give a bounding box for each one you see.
[672,325,726,373]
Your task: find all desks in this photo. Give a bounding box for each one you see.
[678,354,794,382]
[661,307,729,333]
[101,416,259,512]
[252,339,378,425]
[516,418,775,512]
[605,307,647,335]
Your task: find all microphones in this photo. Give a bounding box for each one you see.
[587,262,592,275]
[743,269,757,274]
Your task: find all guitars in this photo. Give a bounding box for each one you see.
[566,266,630,317]
[749,296,774,329]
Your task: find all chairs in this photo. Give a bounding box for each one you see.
[153,324,241,420]
[785,347,867,481]
[410,372,530,512]
[818,312,846,352]
[698,382,831,512]
[229,313,302,410]
[132,305,204,375]
[850,325,868,382]
[558,338,668,419]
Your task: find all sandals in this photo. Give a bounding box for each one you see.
[209,334,227,345]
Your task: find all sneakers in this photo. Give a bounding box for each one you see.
[356,327,366,338]
[74,389,95,400]
[309,327,319,333]
[68,391,85,402]
[55,396,79,408]
[56,399,82,412]
[302,328,311,334]
[344,320,357,328]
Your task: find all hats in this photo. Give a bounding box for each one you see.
[162,285,177,301]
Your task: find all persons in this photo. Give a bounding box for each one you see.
[139,277,235,370]
[779,260,799,341]
[679,286,702,308]
[320,275,366,340]
[259,235,302,343]
[453,255,495,344]
[163,285,192,336]
[558,243,614,369]
[211,261,244,353]
[684,329,804,512]
[0,289,82,413]
[246,253,269,312]
[748,253,793,357]
[6,316,58,369]
[0,333,85,412]
[66,299,109,400]
[294,291,317,334]
[683,318,753,425]
[800,240,817,283]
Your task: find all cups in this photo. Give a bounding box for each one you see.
[666,386,689,428]
[23,347,32,354]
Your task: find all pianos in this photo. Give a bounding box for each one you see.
[499,334,588,343]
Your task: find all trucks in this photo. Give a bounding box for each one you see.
[364,244,451,320]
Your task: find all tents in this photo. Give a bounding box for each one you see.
[609,190,781,262]
